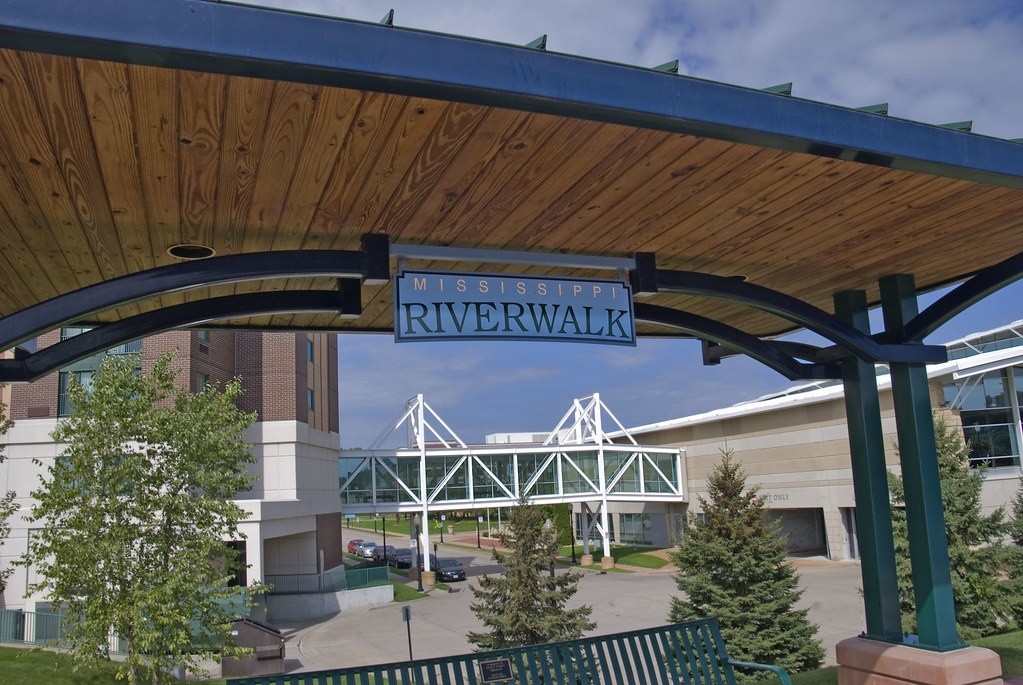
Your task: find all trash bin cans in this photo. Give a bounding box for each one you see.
[447,525,454,535]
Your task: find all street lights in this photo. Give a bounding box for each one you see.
[477,512,483,550]
[440,512,446,544]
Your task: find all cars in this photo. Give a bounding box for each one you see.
[431,558,466,582]
[372,546,395,565]
[356,542,376,558]
[386,548,412,568]
[348,540,363,554]
[418,554,439,571]
